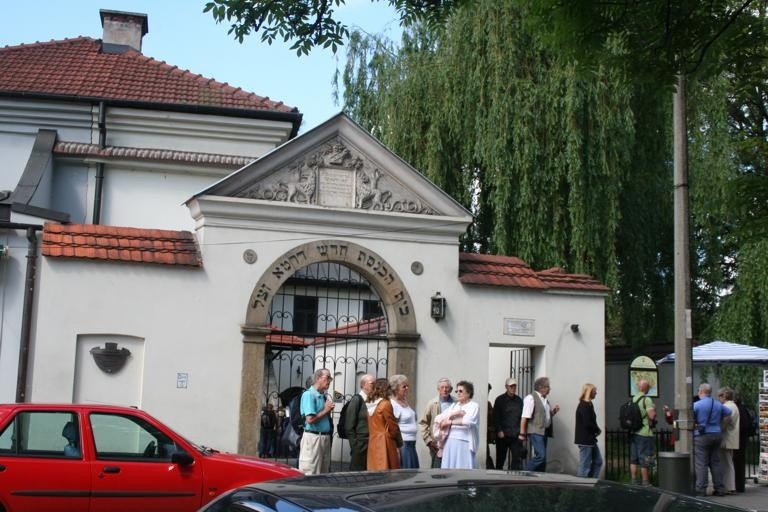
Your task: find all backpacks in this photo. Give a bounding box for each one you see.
[619,396,645,433]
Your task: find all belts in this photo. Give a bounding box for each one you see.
[305,430,330,435]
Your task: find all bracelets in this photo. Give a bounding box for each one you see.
[451,419,453,425]
[519,432,526,436]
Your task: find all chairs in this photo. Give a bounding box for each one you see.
[61,422,81,456]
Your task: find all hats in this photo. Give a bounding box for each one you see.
[506,379,517,385]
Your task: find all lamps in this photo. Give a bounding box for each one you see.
[430,290,447,323]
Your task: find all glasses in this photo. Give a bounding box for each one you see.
[455,390,463,393]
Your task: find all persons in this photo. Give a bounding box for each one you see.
[298,369,338,476]
[418,378,457,468]
[390,373,422,469]
[661,380,749,496]
[363,377,404,471]
[434,380,480,468]
[518,376,559,473]
[573,383,603,478]
[626,379,657,489]
[488,384,495,469]
[343,373,379,470]
[493,379,523,471]
[258,393,299,456]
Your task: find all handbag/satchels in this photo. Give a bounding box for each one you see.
[337,401,351,439]
[291,395,304,436]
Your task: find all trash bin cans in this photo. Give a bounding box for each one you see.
[657,452,690,495]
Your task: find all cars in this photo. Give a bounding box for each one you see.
[1,403,304,510]
[194,468,767,511]
